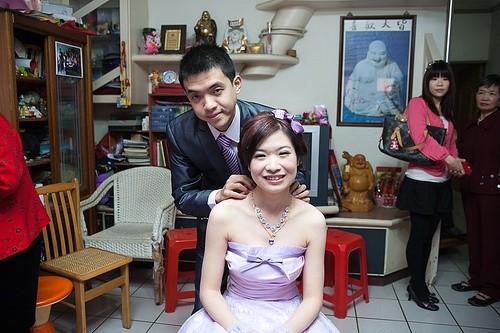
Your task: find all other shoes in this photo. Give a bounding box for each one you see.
[452,279,480,291]
[468,293,500,306]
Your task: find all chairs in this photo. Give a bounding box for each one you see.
[34,178,133,333]
[79,166,177,304]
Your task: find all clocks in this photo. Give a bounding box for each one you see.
[163,70,177,84]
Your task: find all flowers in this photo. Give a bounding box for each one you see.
[299,103,328,126]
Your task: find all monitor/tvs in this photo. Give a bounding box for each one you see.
[301,124,329,207]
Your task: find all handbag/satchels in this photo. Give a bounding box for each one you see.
[379,97,446,165]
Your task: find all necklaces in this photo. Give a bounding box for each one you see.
[478,117,482,121]
[249,192,292,245]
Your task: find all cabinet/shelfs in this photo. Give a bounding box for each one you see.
[62,0,148,106]
[107,89,195,178]
[0,5,96,237]
[95,202,410,277]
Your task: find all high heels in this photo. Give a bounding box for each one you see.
[407,285,439,311]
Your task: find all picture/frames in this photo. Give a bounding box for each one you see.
[336,15,417,126]
[160,24,186,54]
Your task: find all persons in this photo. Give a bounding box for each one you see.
[378,60,465,311]
[343,154,374,193]
[0,111,52,333]
[451,74,500,307]
[195,11,217,45]
[58,48,80,72]
[177,108,341,333]
[170,44,310,316]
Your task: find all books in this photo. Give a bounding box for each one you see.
[153,138,170,167]
[122,139,151,165]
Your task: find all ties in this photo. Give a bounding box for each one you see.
[217,133,240,175]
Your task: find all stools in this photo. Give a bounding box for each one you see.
[31,275,75,333]
[298,229,369,319]
[165,228,197,313]
